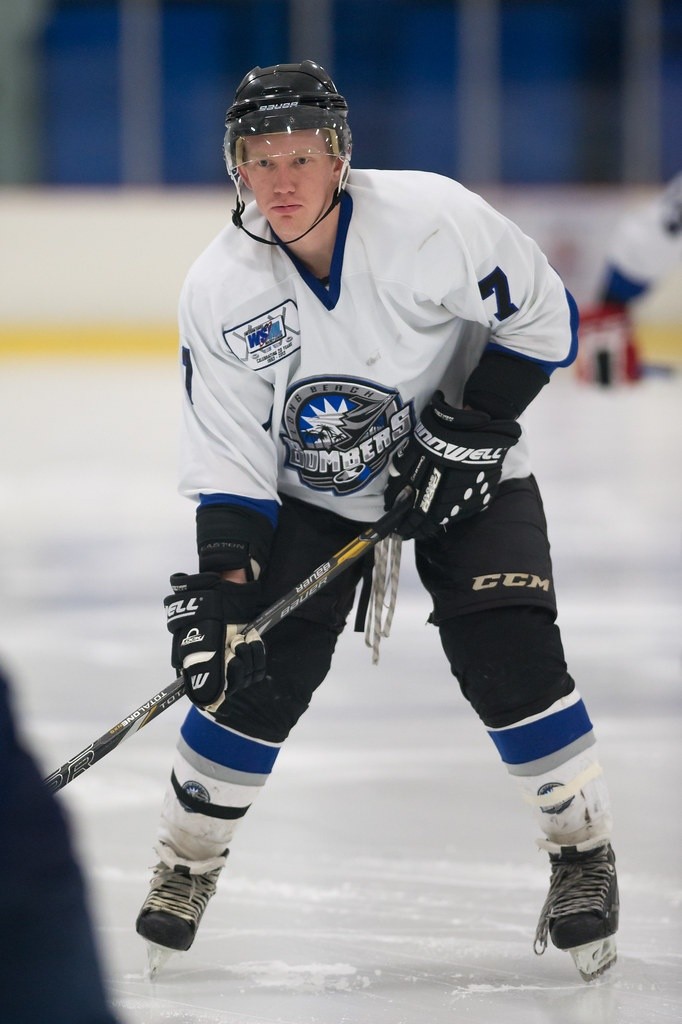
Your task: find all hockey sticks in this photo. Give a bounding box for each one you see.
[43,490,423,798]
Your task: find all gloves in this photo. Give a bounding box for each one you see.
[384,390,522,541]
[164,571,267,713]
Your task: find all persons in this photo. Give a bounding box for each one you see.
[573,175,681,387]
[133,60,621,985]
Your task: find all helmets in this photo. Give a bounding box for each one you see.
[223,60,353,176]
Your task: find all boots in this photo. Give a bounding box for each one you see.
[482,689,620,993]
[136,704,285,982]
